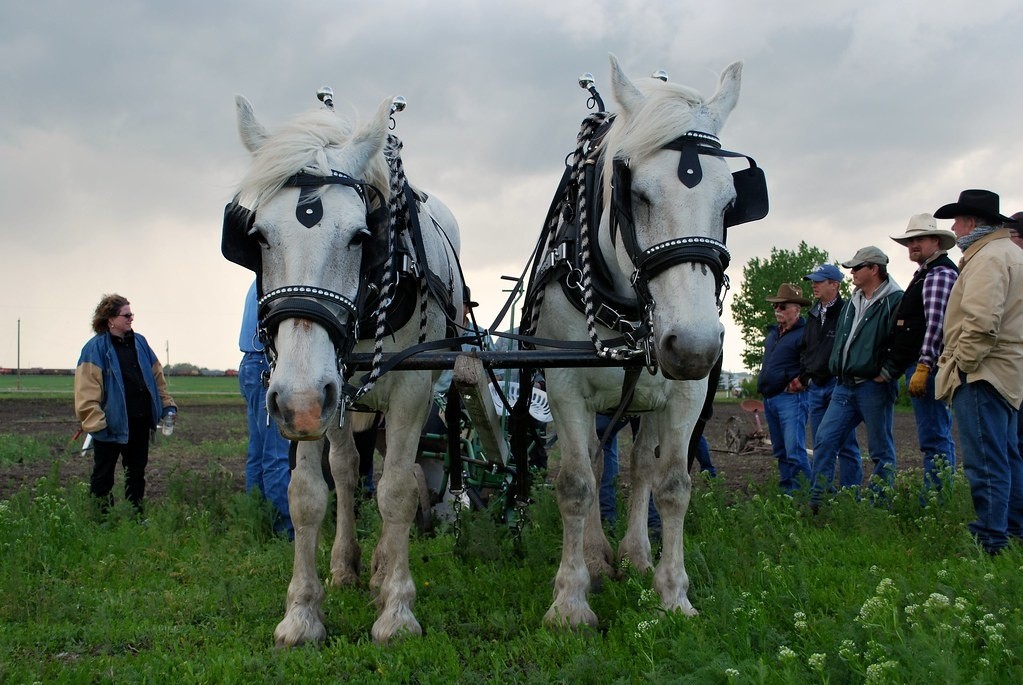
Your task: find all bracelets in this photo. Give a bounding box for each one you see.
[791,379,798,392]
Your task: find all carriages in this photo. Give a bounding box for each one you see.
[220,52,770,646]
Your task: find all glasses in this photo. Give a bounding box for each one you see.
[115,312,132,319]
[853,265,869,271]
[772,303,787,310]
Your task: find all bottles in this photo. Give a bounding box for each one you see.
[162,411,174,436]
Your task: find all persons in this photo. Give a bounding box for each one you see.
[593,409,667,539]
[803,265,863,507]
[324,426,376,523]
[239,277,296,545]
[798,245,907,520]
[758,282,814,498]
[493,325,546,397]
[430,284,495,400]
[933,190,1023,559]
[695,434,718,478]
[885,213,961,520]
[75,294,178,524]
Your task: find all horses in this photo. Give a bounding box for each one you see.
[530,52,743,626]
[234,91,464,646]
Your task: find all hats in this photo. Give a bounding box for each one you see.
[889,213,956,250]
[766,283,812,307]
[841,246,889,269]
[803,264,843,282]
[933,190,1014,223]
